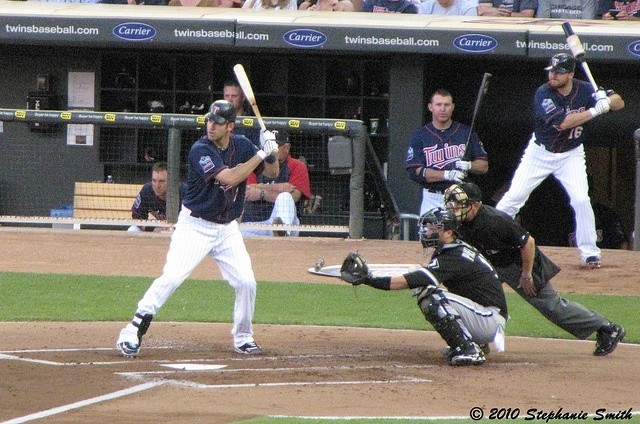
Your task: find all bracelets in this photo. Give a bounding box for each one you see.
[256,149,268,160]
[259,189,265,202]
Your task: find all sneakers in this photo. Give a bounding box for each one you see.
[235,341,265,354]
[444,342,491,355]
[116,328,142,358]
[272,217,288,236]
[452,343,486,366]
[586,257,602,268]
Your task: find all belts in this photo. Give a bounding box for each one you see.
[535,139,563,153]
[429,187,444,193]
[189,210,228,224]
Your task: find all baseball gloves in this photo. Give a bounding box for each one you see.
[341,250,372,286]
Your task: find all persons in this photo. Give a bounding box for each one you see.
[340,207,511,366]
[1,0,639,22]
[237,126,311,237]
[554,169,629,250]
[223,79,261,149]
[128,162,187,233]
[404,89,489,241]
[116,100,281,358]
[443,181,627,357]
[495,53,626,268]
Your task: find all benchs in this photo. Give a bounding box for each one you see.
[73,182,156,218]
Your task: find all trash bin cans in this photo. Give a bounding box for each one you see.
[50,205,73,229]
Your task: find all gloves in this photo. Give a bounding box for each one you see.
[257,139,279,161]
[592,90,612,105]
[259,130,276,148]
[590,100,610,117]
[456,161,471,171]
[444,170,467,184]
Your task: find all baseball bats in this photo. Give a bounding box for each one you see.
[233,64,279,157]
[450,73,493,185]
[562,22,611,114]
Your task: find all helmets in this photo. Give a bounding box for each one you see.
[418,208,462,248]
[445,183,482,223]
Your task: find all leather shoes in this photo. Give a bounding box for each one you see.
[593,321,626,356]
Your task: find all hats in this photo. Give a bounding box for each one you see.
[267,128,291,145]
[545,52,576,74]
[205,100,236,123]
